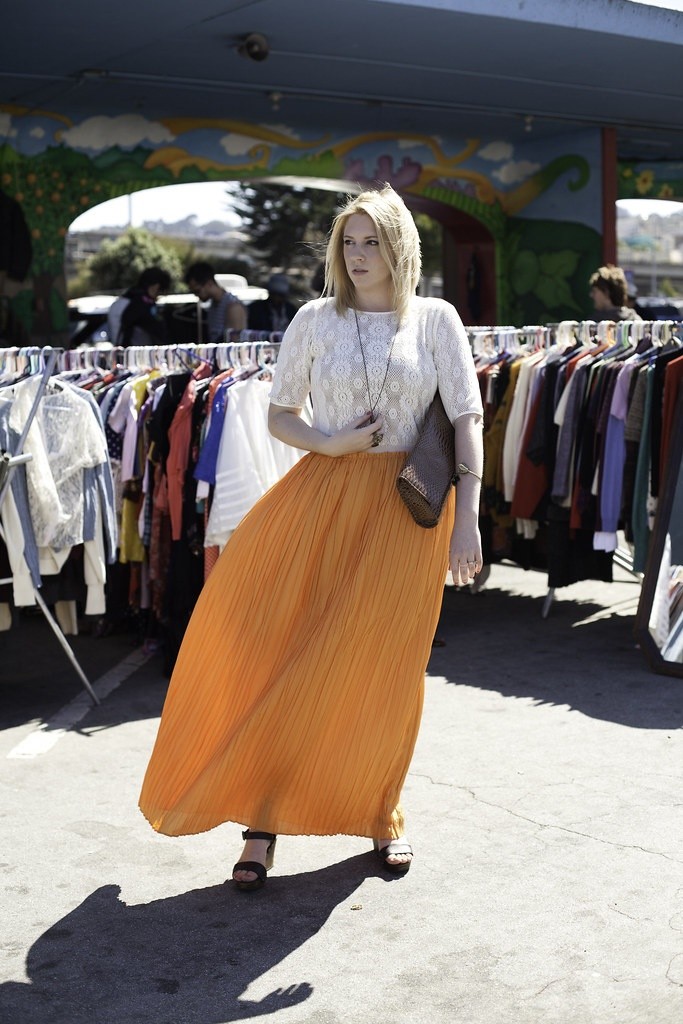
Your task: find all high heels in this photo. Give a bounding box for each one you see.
[231,827,277,888]
[380,843,413,873]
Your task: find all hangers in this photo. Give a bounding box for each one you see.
[0,342,280,396]
[464,319,683,350]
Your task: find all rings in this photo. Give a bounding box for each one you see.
[459,563,468,567]
[371,431,384,448]
[469,561,476,564]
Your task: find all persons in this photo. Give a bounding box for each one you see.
[107,266,172,345]
[589,264,644,320]
[181,262,247,342]
[138,184,485,887]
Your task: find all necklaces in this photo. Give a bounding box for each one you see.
[350,300,400,425]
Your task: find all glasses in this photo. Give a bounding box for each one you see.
[192,281,207,296]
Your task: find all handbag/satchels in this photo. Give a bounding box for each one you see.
[397,389,457,529]
